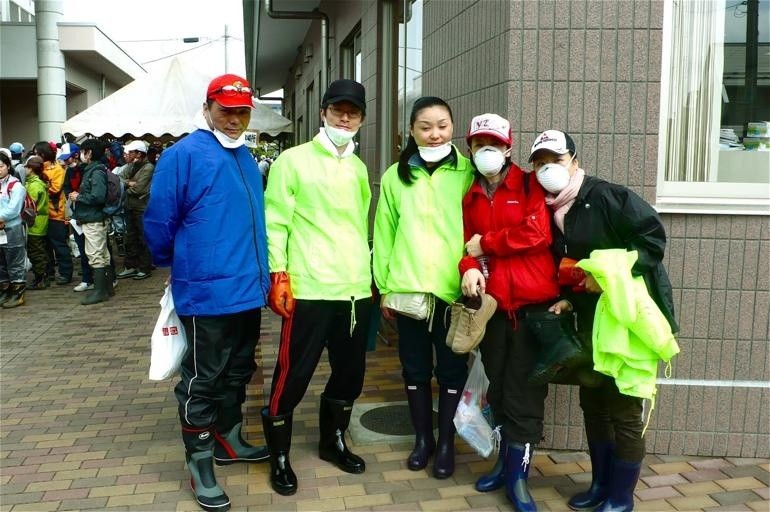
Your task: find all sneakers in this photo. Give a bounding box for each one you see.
[444,294,470,348]
[114,266,137,278]
[73,280,94,292]
[134,269,151,280]
[452,294,496,353]
[27,276,46,291]
[57,273,71,284]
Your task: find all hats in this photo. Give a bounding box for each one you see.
[208,74,256,109]
[9,142,25,154]
[322,79,367,113]
[467,113,514,147]
[0,146,11,159]
[123,141,147,153]
[57,142,77,160]
[528,129,575,163]
[23,156,44,169]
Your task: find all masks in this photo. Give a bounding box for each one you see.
[209,126,247,149]
[78,150,90,162]
[324,121,357,146]
[531,153,577,193]
[466,146,512,178]
[415,142,453,163]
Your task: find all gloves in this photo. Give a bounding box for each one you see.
[271,271,295,319]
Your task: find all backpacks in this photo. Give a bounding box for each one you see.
[6,178,36,227]
[88,167,126,216]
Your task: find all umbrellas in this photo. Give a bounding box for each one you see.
[60,58,292,139]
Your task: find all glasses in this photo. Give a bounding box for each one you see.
[327,105,362,119]
[208,85,255,98]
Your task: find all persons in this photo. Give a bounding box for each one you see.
[20,157,50,290]
[377,97,475,480]
[256,155,268,190]
[0,154,26,307]
[261,79,374,495]
[527,126,681,512]
[147,145,164,166]
[8,142,28,186]
[460,111,561,511]
[69,139,115,305]
[119,141,155,280]
[55,143,119,292]
[33,142,75,283]
[145,75,270,511]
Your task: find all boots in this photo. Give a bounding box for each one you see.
[80,267,109,304]
[568,444,611,510]
[106,265,116,296]
[319,394,366,474]
[2,283,25,308]
[183,428,231,512]
[260,410,298,496]
[434,387,459,477]
[561,313,585,356]
[212,421,268,466]
[505,443,537,512]
[603,458,640,512]
[405,382,433,470]
[476,444,510,492]
[522,311,578,388]
[0,280,10,306]
